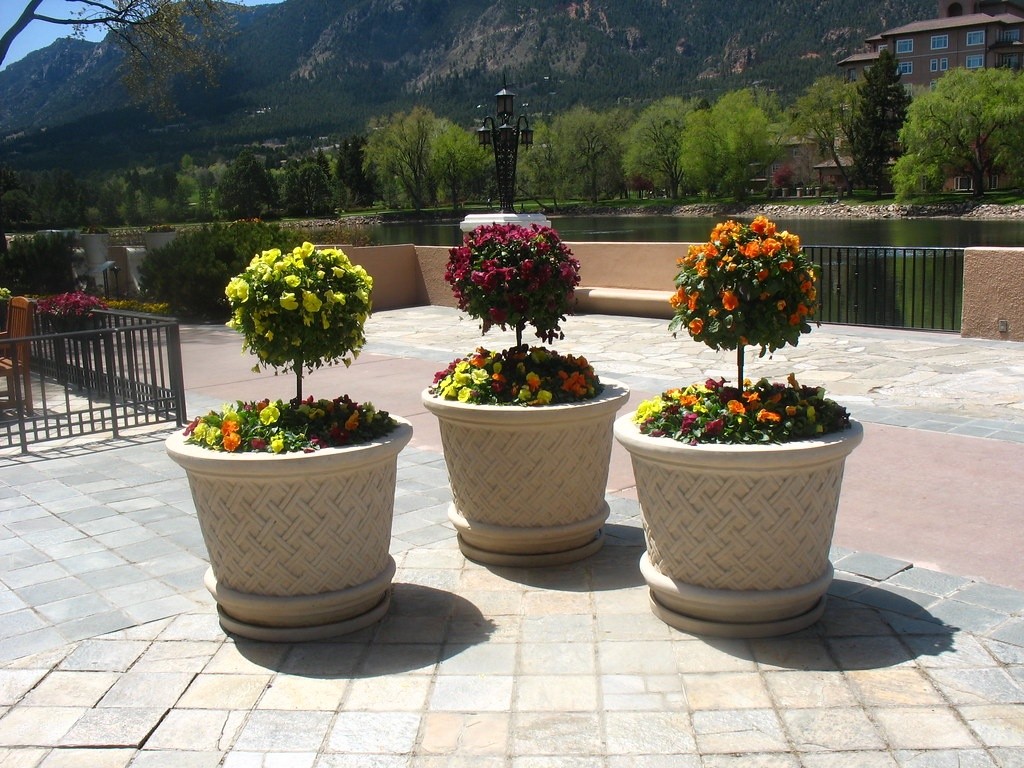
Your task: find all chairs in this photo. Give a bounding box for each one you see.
[0,296,35,418]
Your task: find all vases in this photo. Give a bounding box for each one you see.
[165,414,413,626]
[50,314,95,341]
[421,374,631,555]
[611,410,865,621]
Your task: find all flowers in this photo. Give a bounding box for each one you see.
[182,240,401,454]
[430,221,605,406]
[36,291,109,318]
[632,213,853,446]
